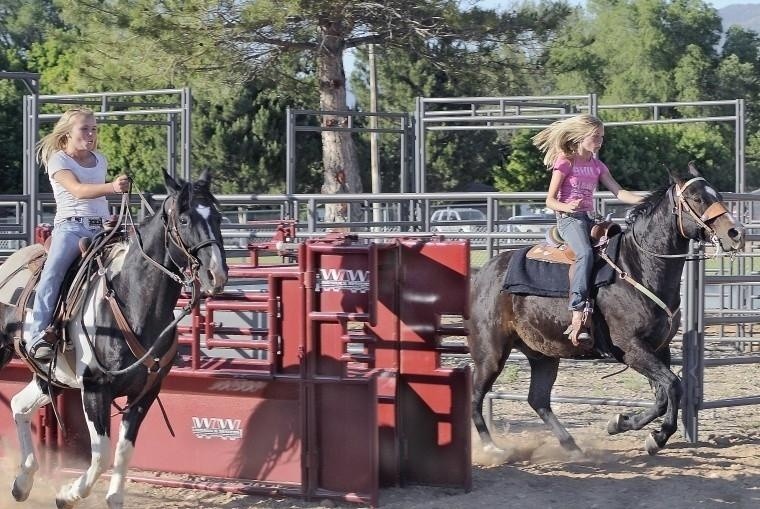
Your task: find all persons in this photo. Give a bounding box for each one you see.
[24,105,132,363]
[528,113,645,351]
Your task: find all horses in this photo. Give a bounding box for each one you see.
[462,160,747,458]
[0,165,229,509]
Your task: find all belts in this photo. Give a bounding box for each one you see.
[555,210,595,221]
[68,215,104,225]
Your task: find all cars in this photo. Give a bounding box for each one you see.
[221,217,239,245]
[430,208,552,233]
[731,190,760,220]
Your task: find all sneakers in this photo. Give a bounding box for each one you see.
[32,338,57,361]
[567,323,592,344]
